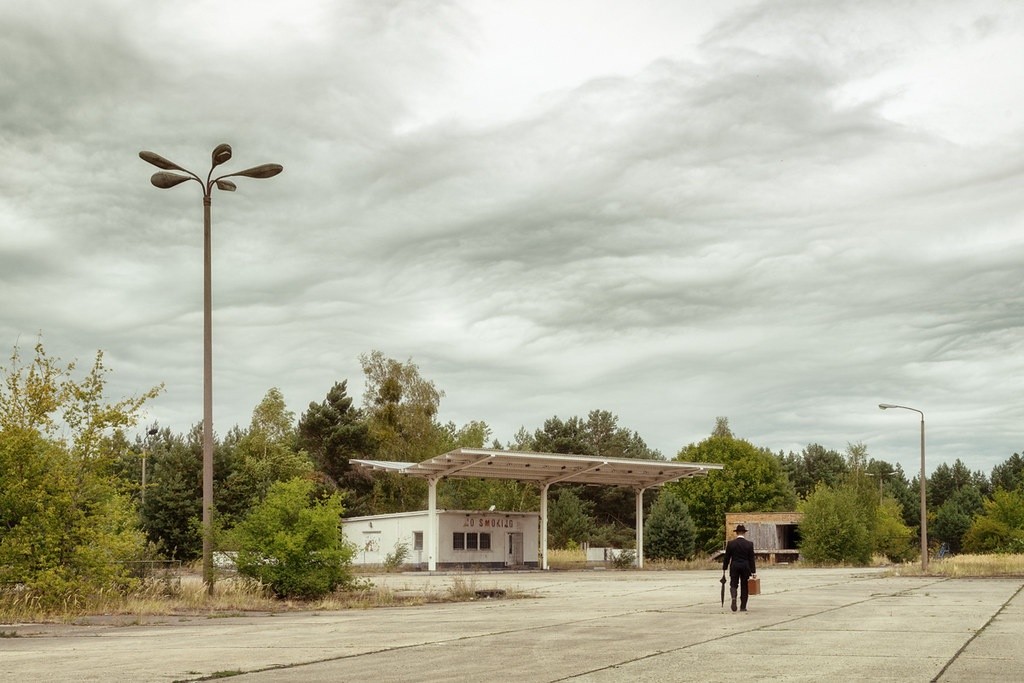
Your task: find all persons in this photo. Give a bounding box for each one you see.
[723,524,757,612]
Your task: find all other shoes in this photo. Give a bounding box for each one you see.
[731,599,737,612]
[740,608,747,611]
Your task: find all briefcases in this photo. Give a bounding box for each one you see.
[748,574,761,595]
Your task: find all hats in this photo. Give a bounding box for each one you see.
[733,525,748,532]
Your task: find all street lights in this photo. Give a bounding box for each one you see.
[142,428,159,507]
[879,403,927,571]
[140,143,284,598]
